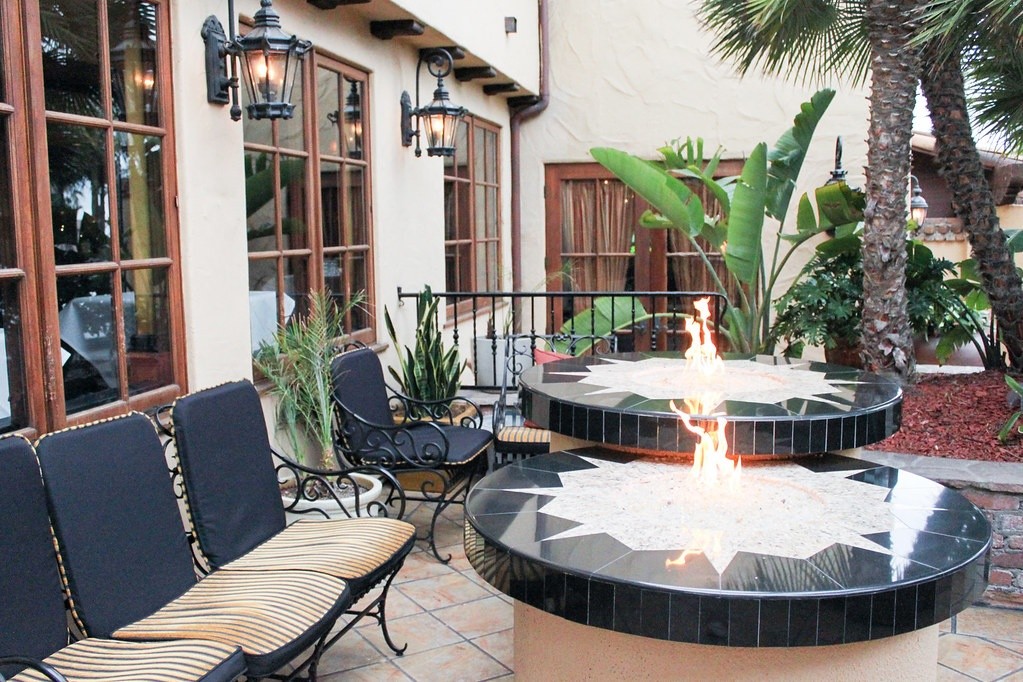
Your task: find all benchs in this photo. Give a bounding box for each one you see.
[0,379,416,682]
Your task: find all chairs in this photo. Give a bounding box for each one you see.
[328,339,494,564]
[493,330,618,472]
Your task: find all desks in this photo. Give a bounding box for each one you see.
[58,291,295,387]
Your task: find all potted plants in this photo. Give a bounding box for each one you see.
[384,284,477,492]
[252,283,383,526]
[468,259,585,385]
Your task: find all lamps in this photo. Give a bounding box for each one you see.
[400,48,468,157]
[94,20,157,128]
[201,0,312,119]
[327,77,361,158]
[910,174,928,237]
